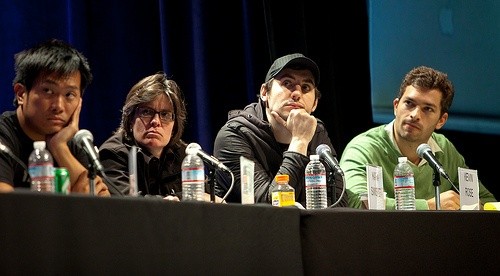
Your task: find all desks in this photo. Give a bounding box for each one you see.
[0,192,500,276]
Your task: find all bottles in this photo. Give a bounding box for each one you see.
[181,148,205,202]
[271,175,295,208]
[28,141,55,193]
[305,155,328,210]
[393,157,416,210]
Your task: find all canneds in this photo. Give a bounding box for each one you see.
[52,167,71,194]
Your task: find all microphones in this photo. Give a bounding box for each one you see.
[73,129,103,172]
[416,143,449,180]
[316,144,344,177]
[185,142,231,174]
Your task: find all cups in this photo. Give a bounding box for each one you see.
[359,192,387,210]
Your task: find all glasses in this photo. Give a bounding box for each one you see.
[137,106,176,123]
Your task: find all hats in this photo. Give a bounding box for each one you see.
[264,53,321,85]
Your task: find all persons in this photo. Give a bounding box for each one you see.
[339,67,497,210]
[214,54,349,208]
[98,73,232,201]
[0,40,111,197]
